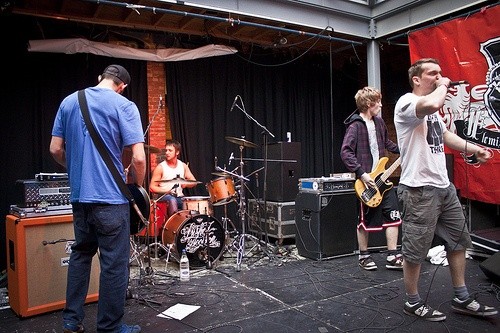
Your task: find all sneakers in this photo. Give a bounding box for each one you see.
[385,253,404,269]
[63,324,84,333]
[452,296,498,316]
[404,299,446,321]
[117,322,142,333]
[359,254,378,270]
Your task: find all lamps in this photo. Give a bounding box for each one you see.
[273,31,288,46]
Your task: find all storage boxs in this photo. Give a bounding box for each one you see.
[247,198,296,239]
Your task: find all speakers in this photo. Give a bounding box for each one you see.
[294,192,359,261]
[5,215,101,318]
[262,142,301,202]
[479,250,500,284]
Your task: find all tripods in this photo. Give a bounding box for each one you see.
[127,104,291,306]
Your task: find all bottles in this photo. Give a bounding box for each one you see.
[180,249,190,278]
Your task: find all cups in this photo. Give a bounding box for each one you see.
[287,132,291,142]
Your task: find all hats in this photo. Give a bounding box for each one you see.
[104,65,130,85]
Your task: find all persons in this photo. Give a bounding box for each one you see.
[341,87,404,269]
[49,65,146,333]
[149,140,196,202]
[394,58,499,321]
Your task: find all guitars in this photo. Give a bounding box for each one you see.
[126,183,151,234]
[354,156,401,208]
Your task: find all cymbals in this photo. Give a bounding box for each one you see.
[211,172,231,177]
[225,136,257,148]
[154,177,203,183]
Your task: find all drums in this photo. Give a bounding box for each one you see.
[180,196,214,218]
[206,176,238,206]
[161,209,226,269]
[136,199,168,238]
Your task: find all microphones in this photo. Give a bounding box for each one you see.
[230,97,238,111]
[450,80,468,84]
